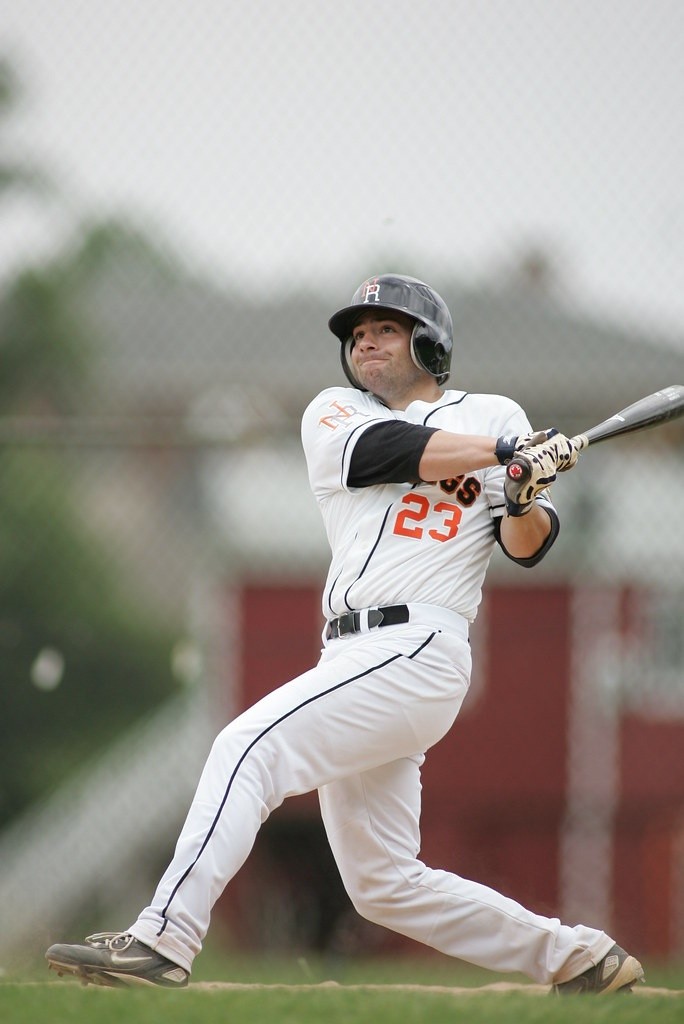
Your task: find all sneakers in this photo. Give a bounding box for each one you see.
[550,944,646,999]
[45,932,189,990]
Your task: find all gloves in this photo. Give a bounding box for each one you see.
[503,431,557,519]
[494,427,578,472]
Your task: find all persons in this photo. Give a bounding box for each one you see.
[45,274,645,998]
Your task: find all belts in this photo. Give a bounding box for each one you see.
[328,605,469,642]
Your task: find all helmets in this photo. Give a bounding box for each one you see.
[327,273,453,392]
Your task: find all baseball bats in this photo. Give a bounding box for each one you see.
[506,384,683,481]
